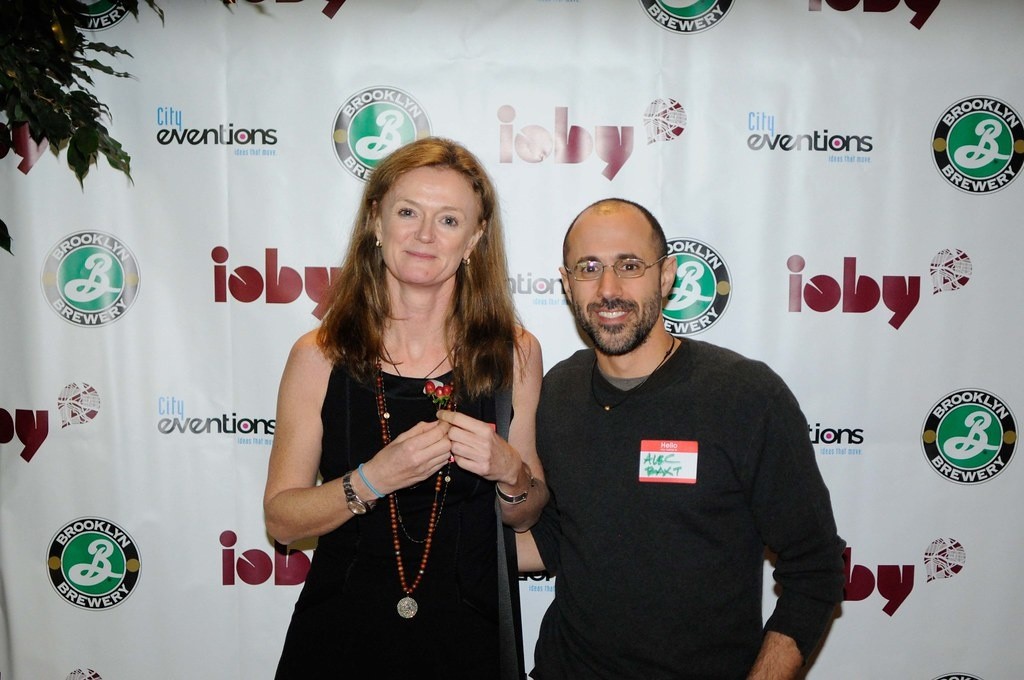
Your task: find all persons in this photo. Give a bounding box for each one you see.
[261,138,542,680]
[515,197,846,680]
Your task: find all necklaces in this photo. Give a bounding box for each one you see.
[375,343,459,620]
[591,333,675,412]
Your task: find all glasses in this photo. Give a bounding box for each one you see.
[565,256,666,281]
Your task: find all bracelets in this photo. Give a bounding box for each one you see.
[495,471,535,505]
[359,464,386,499]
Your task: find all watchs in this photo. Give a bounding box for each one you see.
[342,471,373,516]
